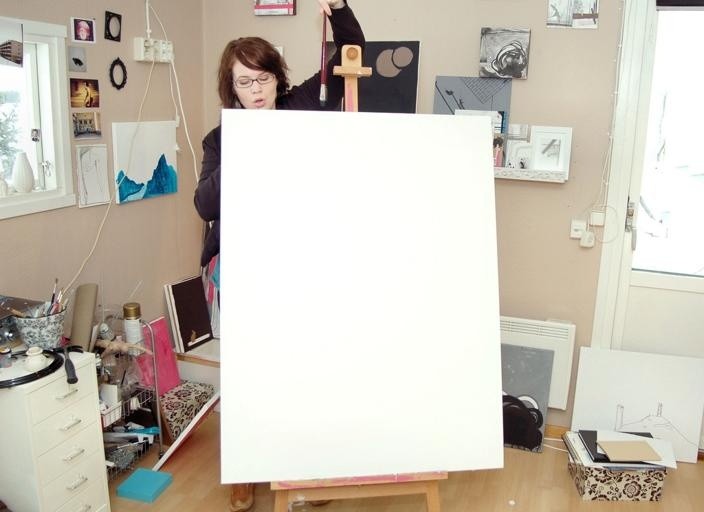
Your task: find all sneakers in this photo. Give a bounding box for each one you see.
[229,484,254,512]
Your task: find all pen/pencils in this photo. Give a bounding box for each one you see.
[47,304,53,315]
[50,304,58,315]
[7,308,31,318]
[58,303,62,313]
[62,299,69,310]
[35,306,40,317]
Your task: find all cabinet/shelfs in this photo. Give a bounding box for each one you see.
[96,316,163,487]
[0,352,111,512]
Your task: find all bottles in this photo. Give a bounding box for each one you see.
[100,324,116,342]
[123,302,143,356]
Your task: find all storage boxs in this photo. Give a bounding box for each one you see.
[566,454,666,503]
[158,378,215,442]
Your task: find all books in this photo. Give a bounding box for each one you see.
[561,429,678,472]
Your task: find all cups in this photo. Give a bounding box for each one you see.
[0,315,16,336]
[0,345,12,368]
[24,347,47,371]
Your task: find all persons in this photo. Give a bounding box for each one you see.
[193,0,366,511]
[84,82,93,109]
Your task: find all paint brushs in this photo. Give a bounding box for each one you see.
[56,287,63,303]
[24,302,34,316]
[57,293,63,304]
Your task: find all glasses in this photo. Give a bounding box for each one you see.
[233,73,274,87]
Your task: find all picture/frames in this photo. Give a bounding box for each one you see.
[528,125,573,176]
